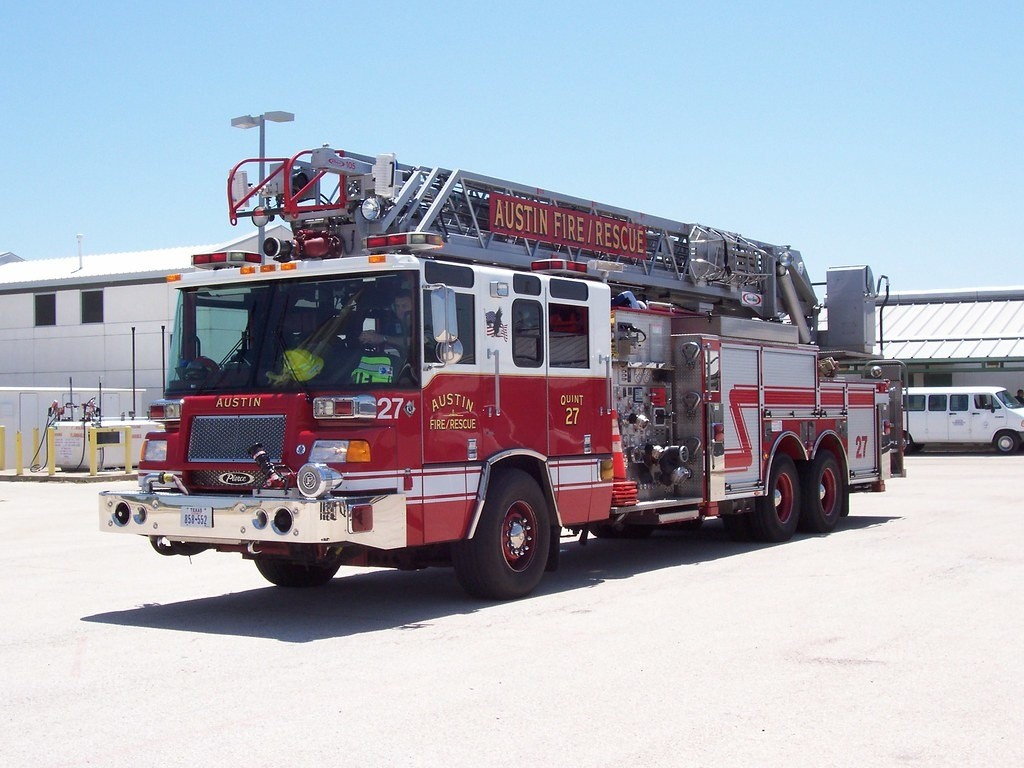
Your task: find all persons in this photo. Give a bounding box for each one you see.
[1015,389,1024,405]
[359,289,434,348]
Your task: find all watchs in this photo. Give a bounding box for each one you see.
[384,335,388,341]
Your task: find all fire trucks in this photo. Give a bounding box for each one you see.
[95,142,909,605]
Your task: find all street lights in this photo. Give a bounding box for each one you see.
[160,325,166,401]
[130,326,136,412]
[230,110,296,260]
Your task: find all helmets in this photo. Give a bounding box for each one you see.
[282,349,327,382]
[185,357,221,390]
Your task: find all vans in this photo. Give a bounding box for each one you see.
[902,386,1024,455]
